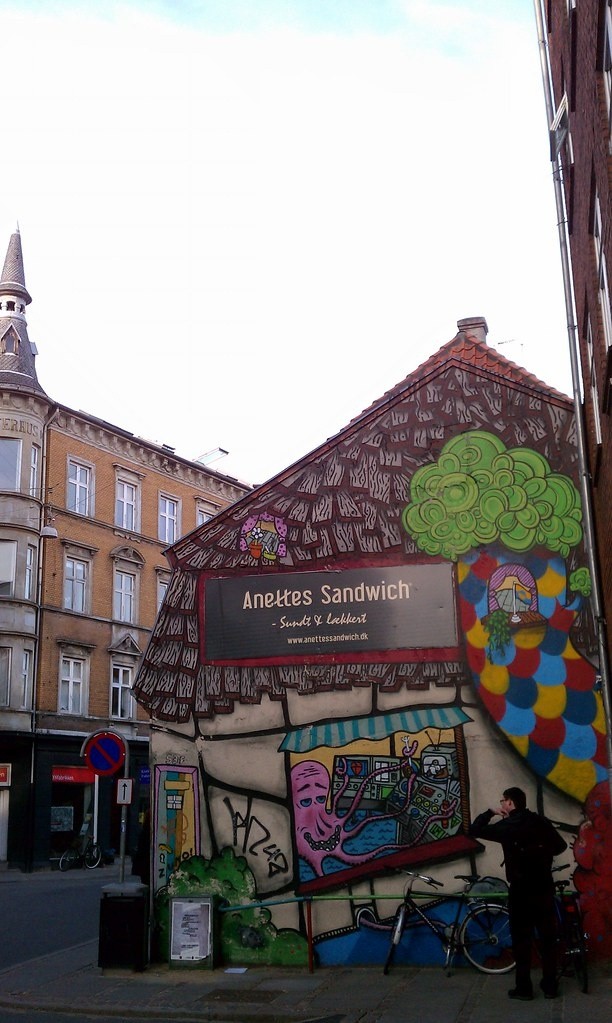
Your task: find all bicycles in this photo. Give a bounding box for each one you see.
[501,858,589,993]
[59,829,102,872]
[382,867,516,975]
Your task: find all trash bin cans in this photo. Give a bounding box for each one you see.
[98,883,150,967]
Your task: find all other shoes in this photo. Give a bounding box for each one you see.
[508,988,534,1001]
[539,983,559,999]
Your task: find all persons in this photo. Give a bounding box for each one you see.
[470,787,568,1000]
[136,808,150,876]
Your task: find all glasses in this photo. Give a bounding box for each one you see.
[499,799,507,805]
